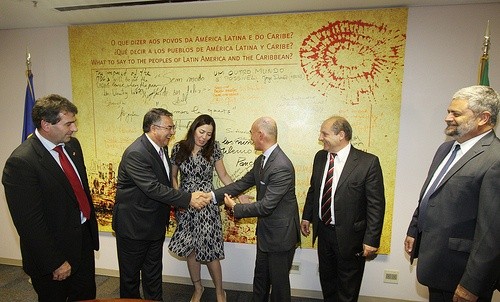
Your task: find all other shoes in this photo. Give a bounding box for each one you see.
[190,287,205,302]
[223,290,227,302]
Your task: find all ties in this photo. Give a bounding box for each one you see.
[158,148,163,162]
[321,152,337,226]
[260,154,266,170]
[52,145,91,221]
[417,144,460,233]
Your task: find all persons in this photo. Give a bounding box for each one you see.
[403,85,500,302]
[111,108,209,302]
[170,114,255,302]
[195,115,301,302]
[2,93,99,301]
[301,115,386,302]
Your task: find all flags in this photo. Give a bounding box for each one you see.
[14,70,36,144]
[477,54,492,88]
